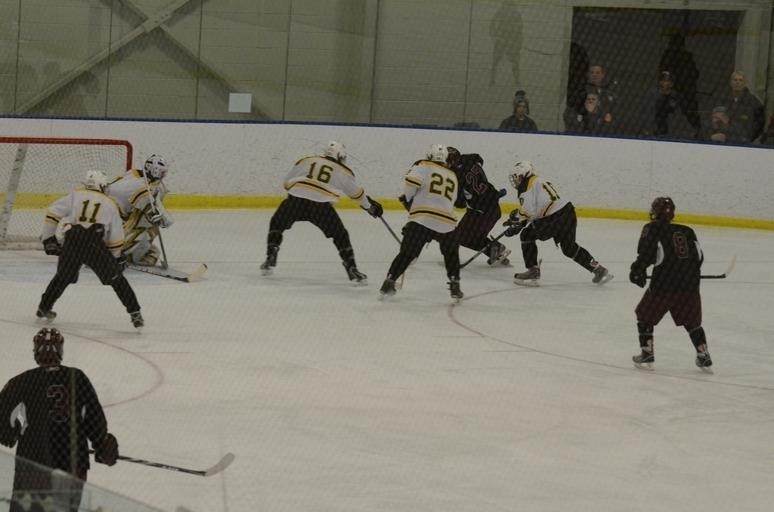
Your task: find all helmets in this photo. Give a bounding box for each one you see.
[32,328,64,367]
[650,196,675,223]
[144,153,168,181]
[509,160,535,189]
[323,140,348,161]
[427,144,460,168]
[83,168,110,191]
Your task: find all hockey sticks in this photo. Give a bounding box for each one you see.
[143,166,169,270]
[91,450,234,477]
[57,249,207,284]
[437,226,514,269]
[634,257,737,279]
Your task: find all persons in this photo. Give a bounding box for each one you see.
[497,90,538,131]
[630,197,715,375]
[101,152,174,266]
[33,169,146,333]
[561,63,765,145]
[0,327,119,512]
[503,161,614,288]
[377,145,469,303]
[442,146,513,270]
[258,141,383,287]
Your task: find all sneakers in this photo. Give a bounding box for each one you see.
[379,278,397,295]
[342,259,368,282]
[696,344,712,366]
[487,235,506,264]
[36,299,56,318]
[260,251,277,270]
[632,341,655,363]
[448,276,465,298]
[131,311,144,328]
[592,259,608,282]
[514,259,542,279]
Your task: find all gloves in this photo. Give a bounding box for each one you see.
[502,208,529,237]
[628,268,648,287]
[43,235,63,256]
[361,195,384,218]
[95,433,119,466]
